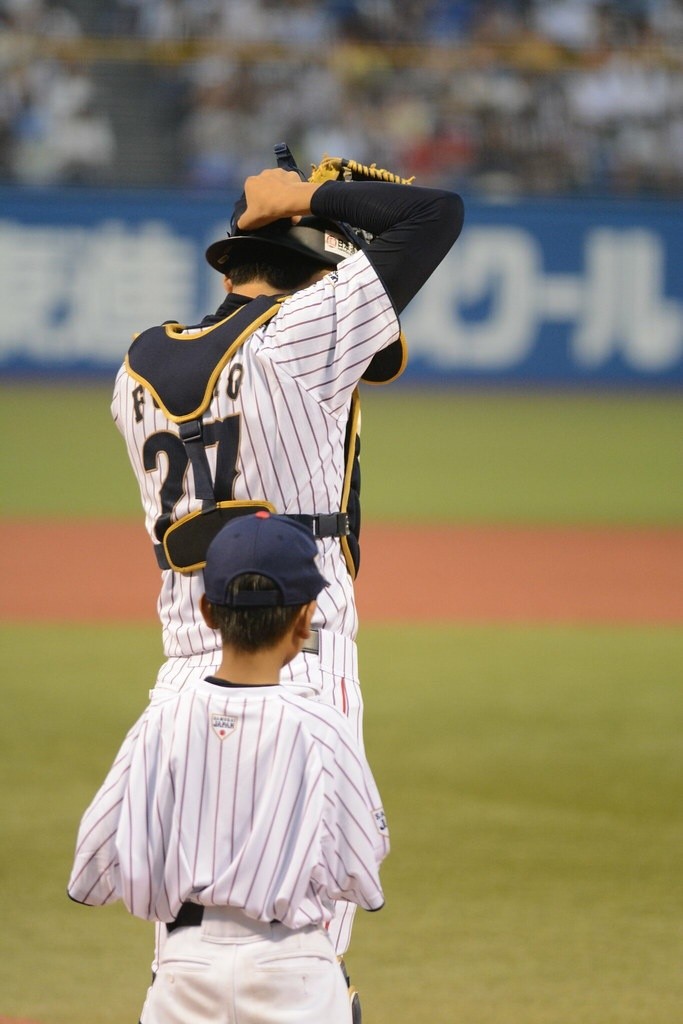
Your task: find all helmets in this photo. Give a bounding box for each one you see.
[205,189,374,274]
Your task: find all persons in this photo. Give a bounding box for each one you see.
[110,143,465,1024]
[0,0,682,200]
[67,512,390,1024]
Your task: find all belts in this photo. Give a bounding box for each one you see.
[302,629,319,654]
[166,902,281,933]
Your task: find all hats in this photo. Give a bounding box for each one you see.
[203,510,326,608]
